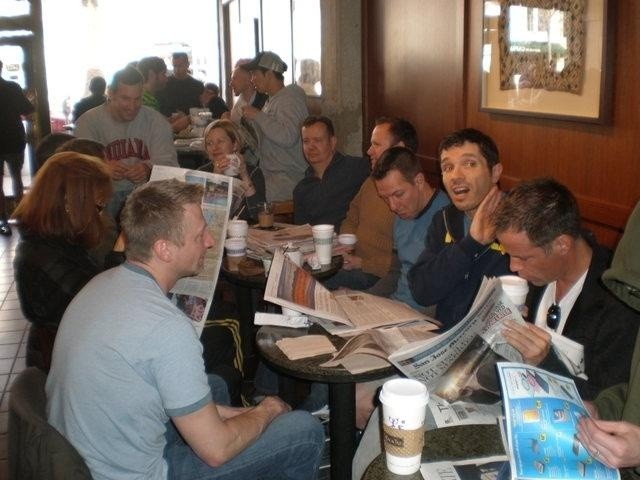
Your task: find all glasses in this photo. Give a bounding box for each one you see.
[546,303,561,333]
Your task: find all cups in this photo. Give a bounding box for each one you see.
[499,275,527,319]
[227,220,249,238]
[312,224,333,266]
[222,154,241,175]
[224,238,248,272]
[379,379,428,475]
[257,202,275,230]
[338,234,356,263]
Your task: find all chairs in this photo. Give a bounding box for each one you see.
[9,367,94,480]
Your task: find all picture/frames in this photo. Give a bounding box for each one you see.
[477,0,618,126]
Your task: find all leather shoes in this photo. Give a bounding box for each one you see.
[2,219,12,236]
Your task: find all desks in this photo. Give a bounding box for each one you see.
[221,253,344,309]
[255,304,441,480]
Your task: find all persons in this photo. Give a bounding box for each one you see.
[351,177,639,475]
[221,59,268,122]
[575,199,638,478]
[75,66,177,228]
[179,84,219,115]
[34,132,75,171]
[206,97,231,120]
[73,77,108,122]
[1,62,36,237]
[253,115,418,405]
[355,128,518,430]
[240,51,310,202]
[297,58,321,96]
[45,178,324,479]
[196,120,266,224]
[157,52,205,115]
[57,139,109,162]
[139,56,191,133]
[19,87,50,148]
[338,145,451,320]
[293,115,372,235]
[14,152,250,408]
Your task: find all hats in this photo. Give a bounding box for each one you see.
[241,51,288,72]
